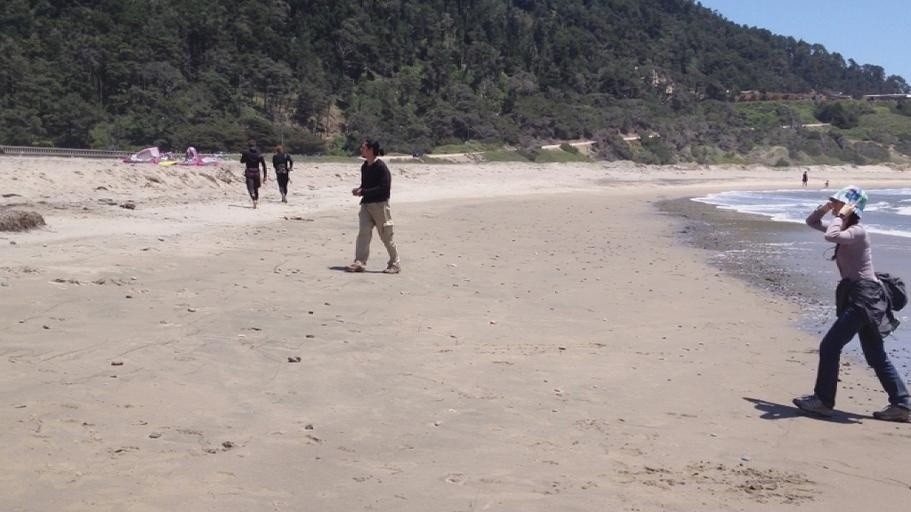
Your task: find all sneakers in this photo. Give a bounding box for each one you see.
[345,265,365,272]
[384,267,400,273]
[874,405,911,423]
[793,395,832,416]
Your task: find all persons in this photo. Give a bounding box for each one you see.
[239,138,267,210]
[345,138,403,274]
[271,145,293,204]
[802,171,809,187]
[791,185,911,424]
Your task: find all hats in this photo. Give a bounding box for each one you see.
[830,187,867,219]
